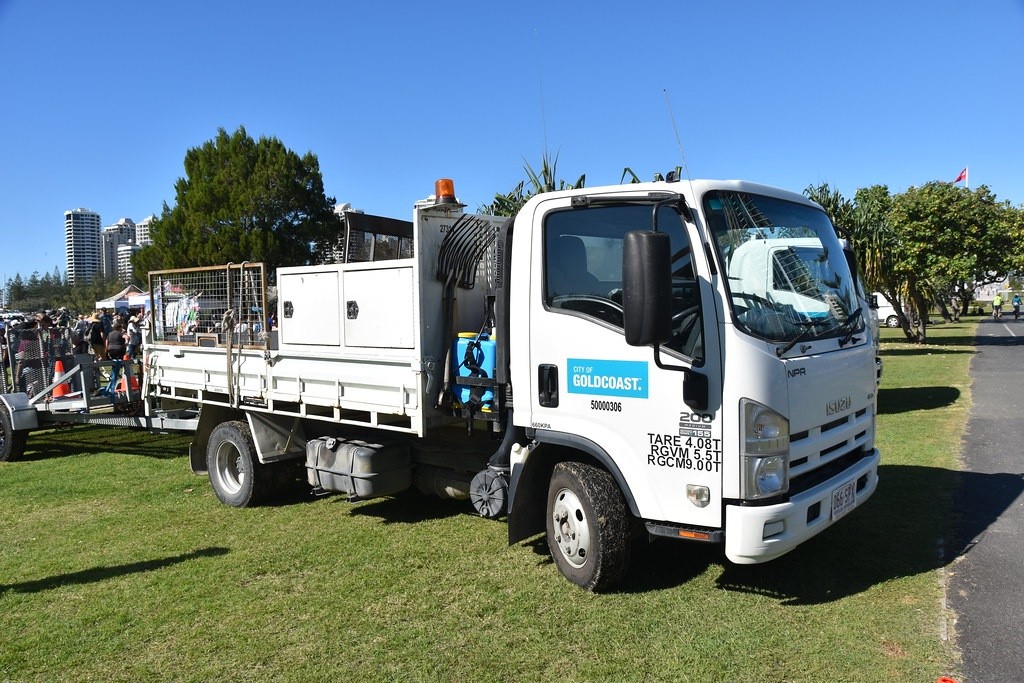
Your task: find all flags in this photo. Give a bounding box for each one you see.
[954,167,968,183]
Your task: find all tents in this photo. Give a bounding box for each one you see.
[95,284,230,325]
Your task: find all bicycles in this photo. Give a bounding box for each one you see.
[1013,303,1022,320]
[992,304,1003,321]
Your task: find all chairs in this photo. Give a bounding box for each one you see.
[548,236,606,300]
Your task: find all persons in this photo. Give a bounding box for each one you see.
[1012,293,1023,317]
[234,315,260,334]
[993,293,1003,317]
[0,305,145,396]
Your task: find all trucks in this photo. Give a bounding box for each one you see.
[140,168,881,595]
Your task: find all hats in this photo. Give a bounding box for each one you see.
[130,316,140,323]
[1014,293,1018,296]
[87,313,102,322]
[58,307,67,312]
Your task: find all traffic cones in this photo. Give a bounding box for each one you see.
[115,355,141,394]
[50,360,81,403]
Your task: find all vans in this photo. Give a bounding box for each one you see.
[870,291,910,328]
